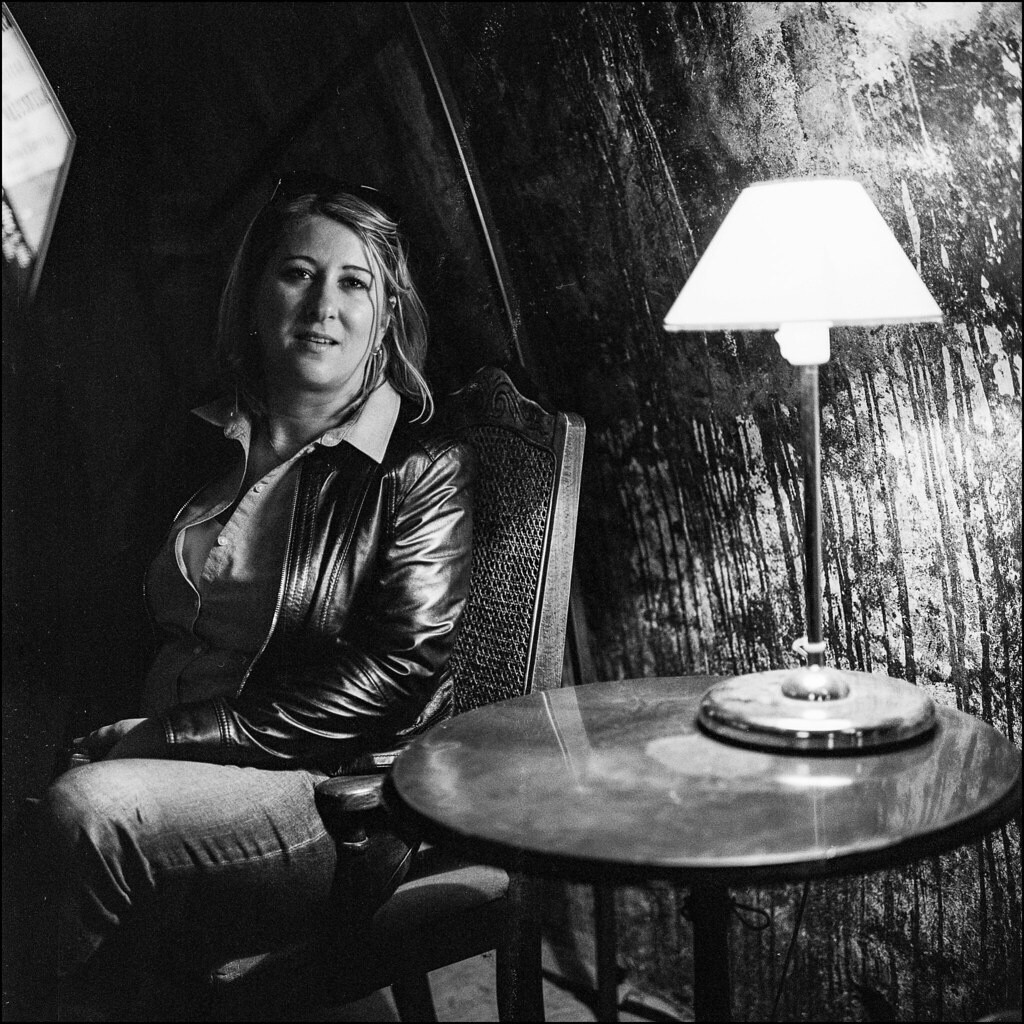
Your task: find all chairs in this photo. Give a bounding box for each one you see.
[83,365,586,1022]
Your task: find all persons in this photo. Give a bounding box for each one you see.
[1,181,474,1024]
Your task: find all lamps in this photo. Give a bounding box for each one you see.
[662,176,943,759]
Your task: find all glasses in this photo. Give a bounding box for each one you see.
[270,171,412,239]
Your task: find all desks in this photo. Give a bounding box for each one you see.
[382,672,1024,1024]
